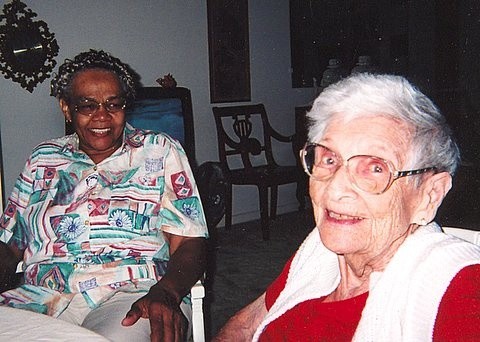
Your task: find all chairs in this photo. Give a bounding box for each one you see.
[214,104,311,240]
[14,121,205,342]
[66,74,232,234]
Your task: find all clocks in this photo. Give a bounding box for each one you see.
[0,0,59,94]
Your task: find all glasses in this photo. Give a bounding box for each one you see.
[299,143,437,195]
[69,96,128,116]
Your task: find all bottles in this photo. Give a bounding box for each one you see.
[317,59,349,98]
[350,55,379,75]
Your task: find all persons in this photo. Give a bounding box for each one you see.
[0,50,210,342]
[212,73,480,342]
